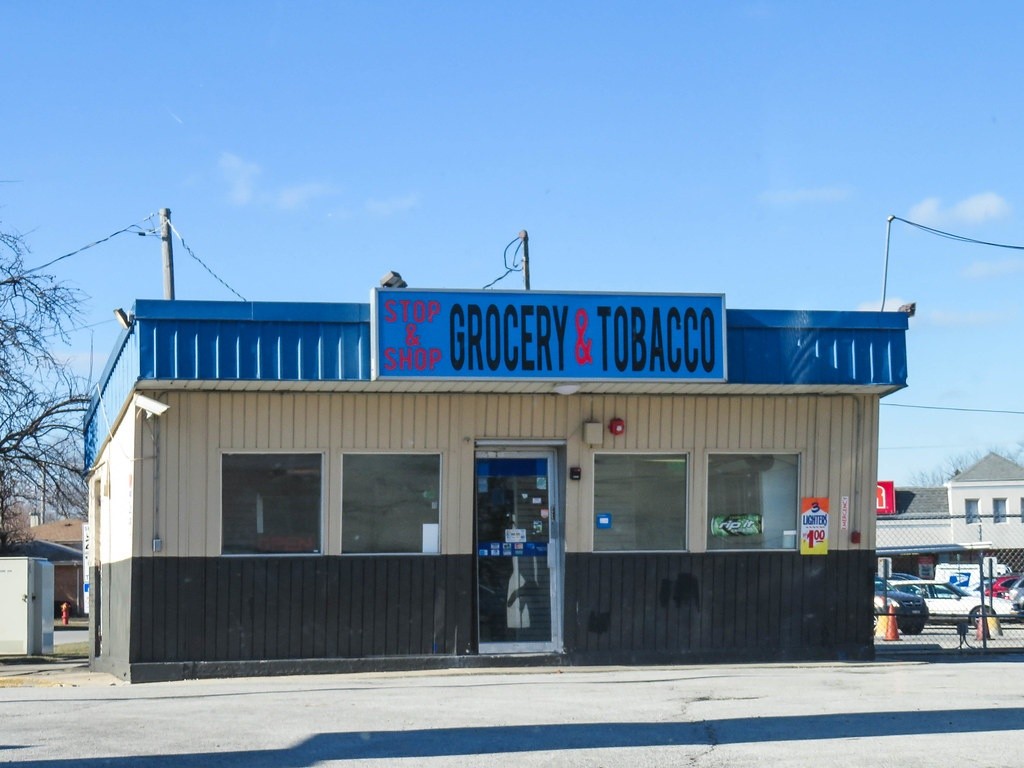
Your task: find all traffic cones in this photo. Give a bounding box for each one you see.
[973,608,986,642]
[882,603,904,641]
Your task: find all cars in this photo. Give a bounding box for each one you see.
[886,571,1024,625]
[873,573,929,635]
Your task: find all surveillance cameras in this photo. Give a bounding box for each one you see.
[135,395,170,417]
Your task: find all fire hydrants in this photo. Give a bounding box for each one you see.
[60,602,72,625]
